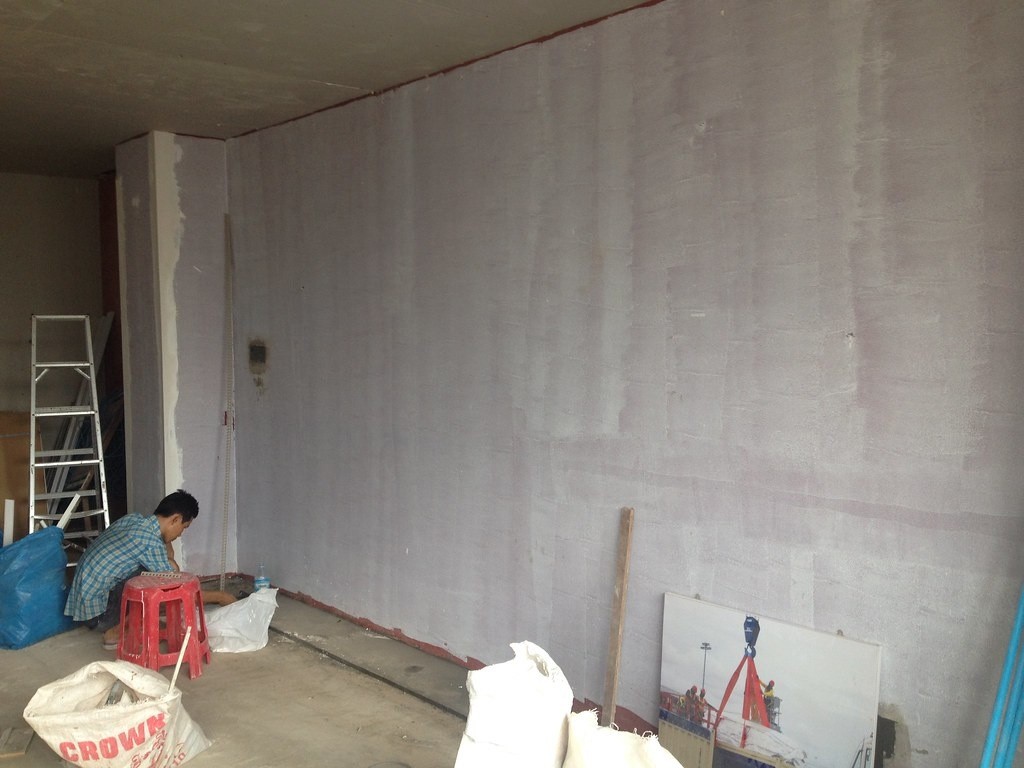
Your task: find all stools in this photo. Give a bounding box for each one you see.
[116,572,214,679]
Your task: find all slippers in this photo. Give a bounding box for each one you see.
[102,633,163,650]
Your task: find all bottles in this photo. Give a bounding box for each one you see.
[254,562,271,591]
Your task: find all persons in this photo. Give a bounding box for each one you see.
[673,678,776,731]
[62,488,240,651]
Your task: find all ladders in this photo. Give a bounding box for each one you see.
[27,313,115,570]
